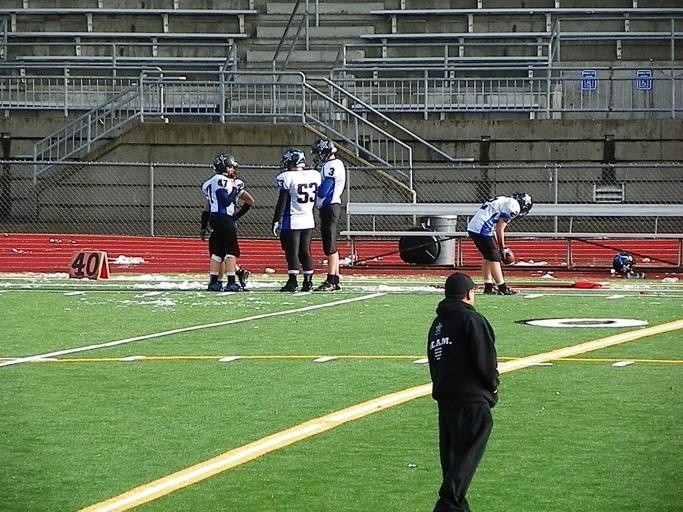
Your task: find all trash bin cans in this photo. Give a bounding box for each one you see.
[420,216,458,266]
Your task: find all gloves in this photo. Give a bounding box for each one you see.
[232,218,239,232]
[500,248,510,261]
[273,222,280,236]
[199,229,207,241]
[491,393,500,404]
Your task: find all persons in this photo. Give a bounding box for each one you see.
[421,272,502,512]
[268,147,322,293]
[206,154,242,292]
[309,137,347,295]
[198,153,254,288]
[464,191,534,297]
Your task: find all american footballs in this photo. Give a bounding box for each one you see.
[503,248,514,265]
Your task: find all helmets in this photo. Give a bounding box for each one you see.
[512,193,532,217]
[281,149,306,172]
[613,254,633,274]
[310,139,336,166]
[212,154,239,176]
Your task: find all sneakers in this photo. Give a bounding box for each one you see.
[208,271,249,291]
[276,286,298,293]
[300,282,342,293]
[485,283,518,295]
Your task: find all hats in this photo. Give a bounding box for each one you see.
[445,272,475,294]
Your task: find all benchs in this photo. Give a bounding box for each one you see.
[339,200,683,267]
[355,1,682,92]
[0,0,258,87]
